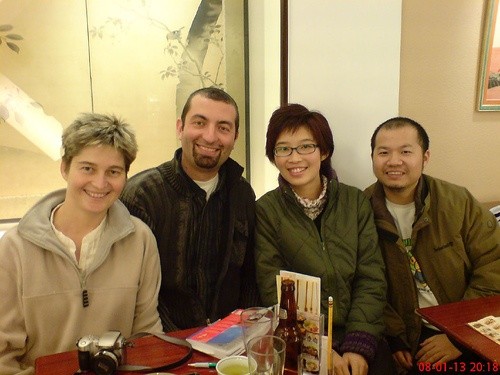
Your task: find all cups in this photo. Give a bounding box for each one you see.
[240,306,274,356]
[246,335,286,375]
[216,356,258,375]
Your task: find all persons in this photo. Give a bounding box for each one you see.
[0,112,162,375]
[255,103,388,375]
[362,117,500,375]
[118,88,262,333]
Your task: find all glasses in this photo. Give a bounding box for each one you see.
[274,144,318,157]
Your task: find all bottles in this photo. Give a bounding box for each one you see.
[272,278,305,375]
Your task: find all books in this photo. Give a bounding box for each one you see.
[186,309,278,359]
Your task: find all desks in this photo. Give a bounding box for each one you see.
[33,325,299,375]
[415,294,500,371]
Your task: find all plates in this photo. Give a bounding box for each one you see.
[473,315,500,345]
[299,314,320,374]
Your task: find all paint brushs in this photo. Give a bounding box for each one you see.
[327,295,335,370]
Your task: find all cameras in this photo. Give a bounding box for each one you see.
[73,330,129,375]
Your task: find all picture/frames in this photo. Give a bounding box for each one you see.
[475,0,500,111]
[0,0,250,223]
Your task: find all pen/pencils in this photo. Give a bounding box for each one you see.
[187,362,217,369]
